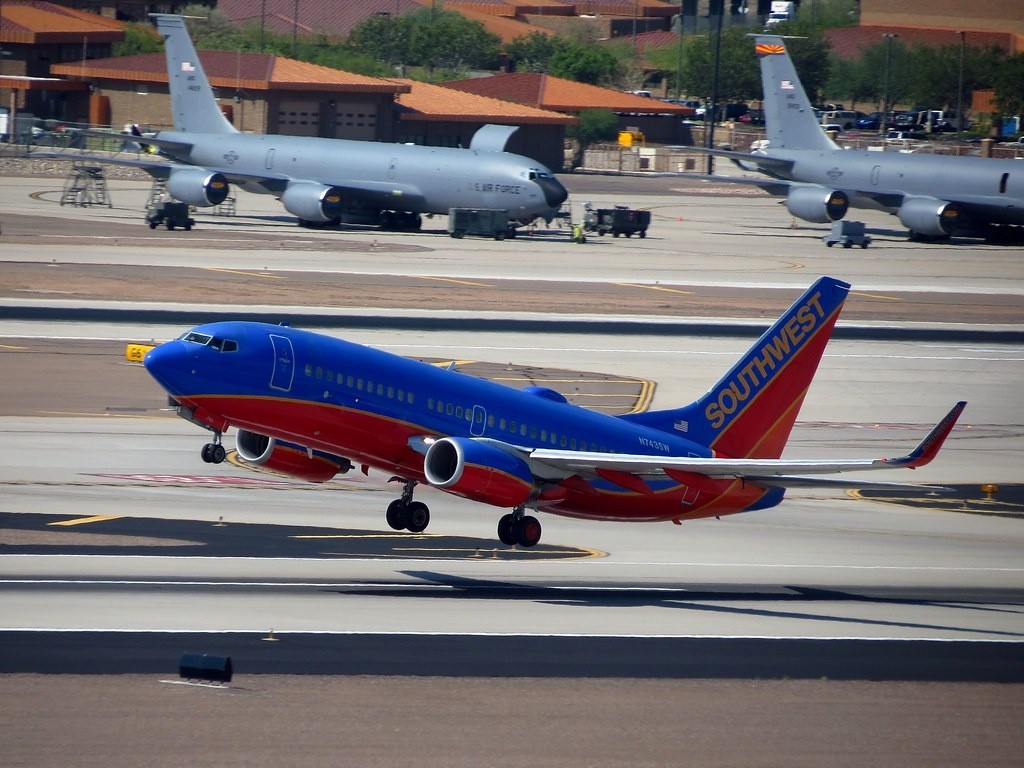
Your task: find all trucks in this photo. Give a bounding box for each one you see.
[766,1,795,30]
[149,202,195,231]
[822,220,871,249]
[448,208,515,241]
[582,201,651,238]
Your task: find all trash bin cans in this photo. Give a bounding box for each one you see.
[103,130,128,152]
[86,128,113,151]
[825,130,839,141]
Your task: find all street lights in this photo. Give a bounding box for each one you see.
[958,31,965,141]
[881,34,898,147]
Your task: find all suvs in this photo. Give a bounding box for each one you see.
[810,103,963,131]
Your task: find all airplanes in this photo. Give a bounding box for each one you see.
[0,13,568,240]
[664,34,1024,244]
[145,278,967,548]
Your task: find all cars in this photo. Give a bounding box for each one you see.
[626,91,765,126]
[885,132,930,145]
